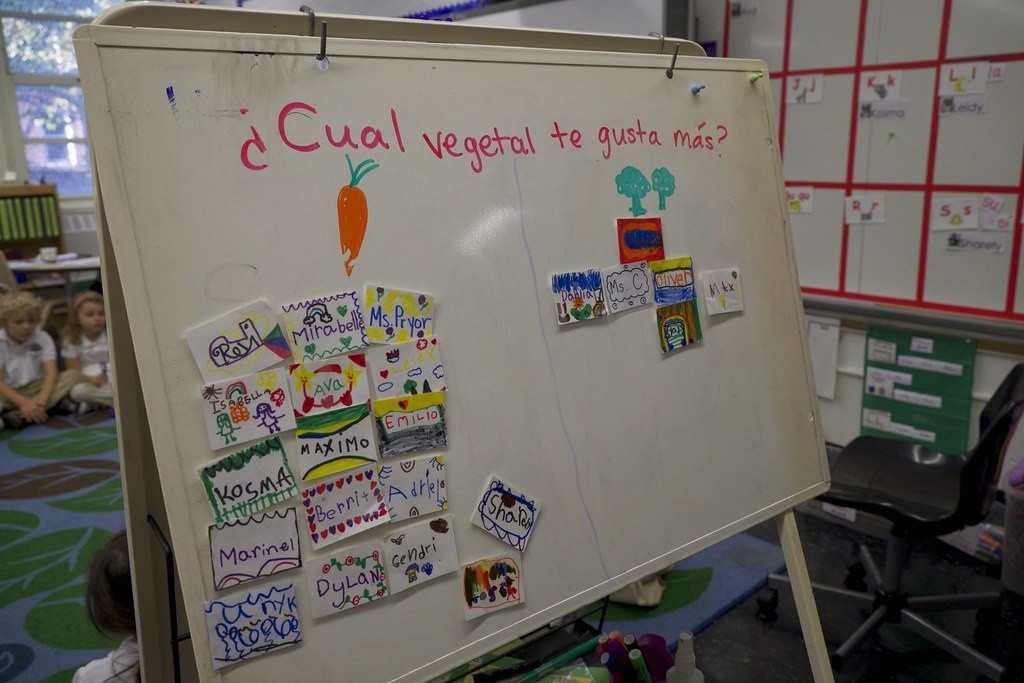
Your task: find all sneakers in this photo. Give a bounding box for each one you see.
[4,408,25,430]
[56,397,76,413]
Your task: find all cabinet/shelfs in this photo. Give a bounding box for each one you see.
[0,180,74,342]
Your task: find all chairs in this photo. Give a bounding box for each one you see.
[760,362,1022,674]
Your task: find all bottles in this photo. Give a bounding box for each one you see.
[666,630,706,683]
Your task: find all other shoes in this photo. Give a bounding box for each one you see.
[76,401,97,416]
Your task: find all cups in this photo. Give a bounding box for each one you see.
[40,247,58,263]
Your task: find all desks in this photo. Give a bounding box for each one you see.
[7,252,104,327]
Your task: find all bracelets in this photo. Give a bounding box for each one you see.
[32,400,48,410]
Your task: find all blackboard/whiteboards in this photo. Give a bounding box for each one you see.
[72,24,834,683]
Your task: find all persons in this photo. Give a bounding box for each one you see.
[0,289,79,432]
[69,524,144,683]
[58,288,116,415]
